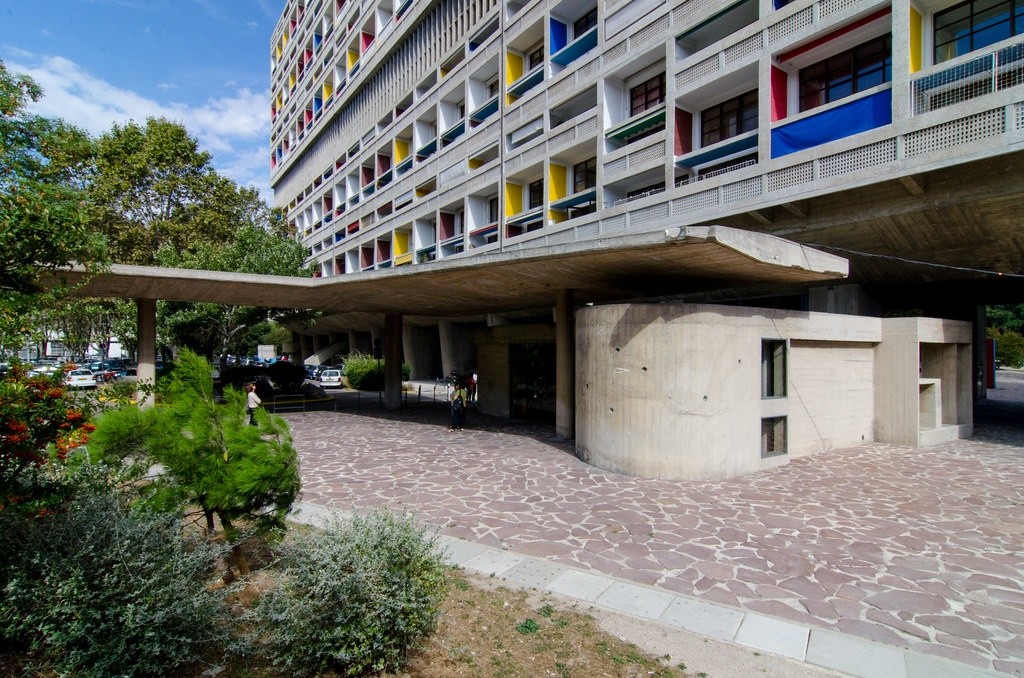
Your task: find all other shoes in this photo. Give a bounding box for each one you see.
[449,429,454,432]
[459,428,463,431]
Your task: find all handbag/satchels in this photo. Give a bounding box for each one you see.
[453,389,463,410]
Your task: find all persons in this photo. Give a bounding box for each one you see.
[448,370,477,432]
[248,383,261,428]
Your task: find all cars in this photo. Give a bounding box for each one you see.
[64,368,96,389]
[319,369,345,389]
[304,364,334,381]
[219,354,292,368]
[0,358,164,383]
